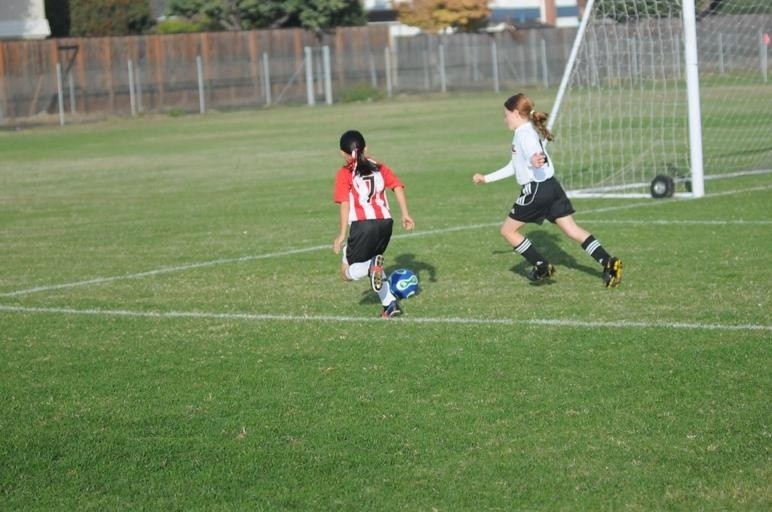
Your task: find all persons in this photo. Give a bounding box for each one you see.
[333,130,415,318]
[472,92,623,289]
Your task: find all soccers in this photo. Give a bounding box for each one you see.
[389,268,418,299]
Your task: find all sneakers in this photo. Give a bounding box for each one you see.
[602,256,623,289]
[527,261,555,282]
[380,300,402,318]
[368,253,385,293]
